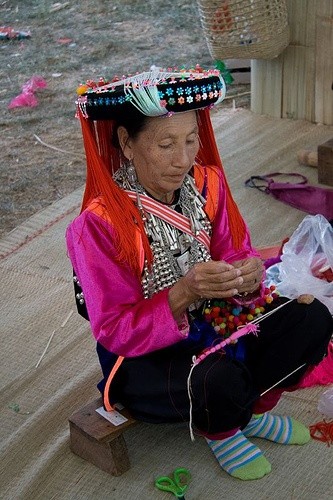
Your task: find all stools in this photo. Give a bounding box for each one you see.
[68,397,141,478]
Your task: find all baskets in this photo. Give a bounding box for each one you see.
[196,0,291,61]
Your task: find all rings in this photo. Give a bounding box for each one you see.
[254,278,257,283]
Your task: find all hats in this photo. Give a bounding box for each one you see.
[76,64,228,121]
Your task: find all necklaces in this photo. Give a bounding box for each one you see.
[112,163,212,312]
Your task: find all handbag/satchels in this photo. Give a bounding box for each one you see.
[246,171,333,221]
[262,214,333,320]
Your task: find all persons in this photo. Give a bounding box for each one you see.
[66,71,333,480]
